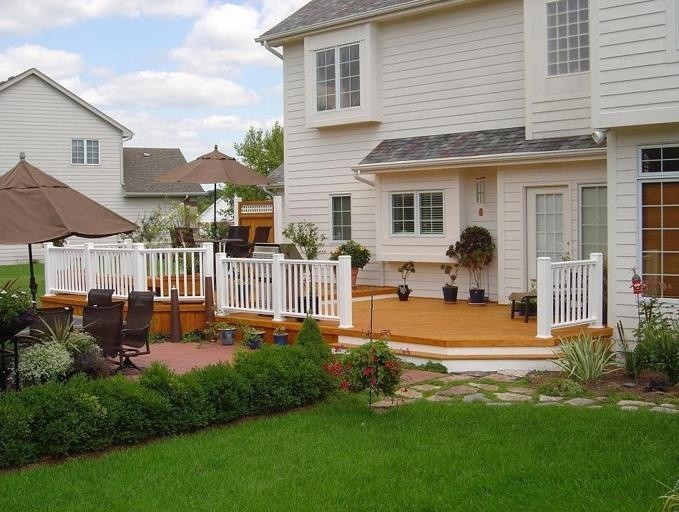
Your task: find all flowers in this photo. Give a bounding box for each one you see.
[323,329,412,398]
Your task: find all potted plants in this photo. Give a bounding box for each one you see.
[204,319,289,350]
[281,219,329,322]
[396,260,416,301]
[441,225,497,307]
[328,240,372,290]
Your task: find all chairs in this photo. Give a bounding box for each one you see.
[509,291,537,323]
[0,289,155,393]
[169,225,273,273]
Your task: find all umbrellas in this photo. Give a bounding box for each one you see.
[0,149,142,304]
[154,142,279,239]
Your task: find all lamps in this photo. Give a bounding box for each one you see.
[591,130,609,143]
